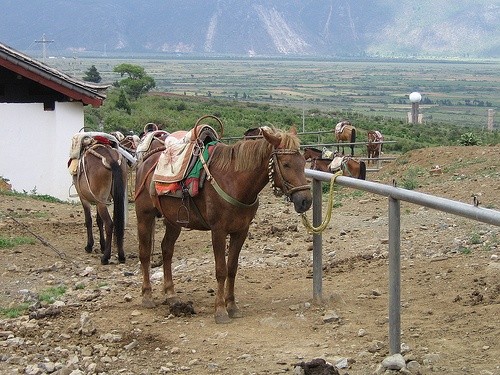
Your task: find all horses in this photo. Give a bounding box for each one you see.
[73,120,384,324]
